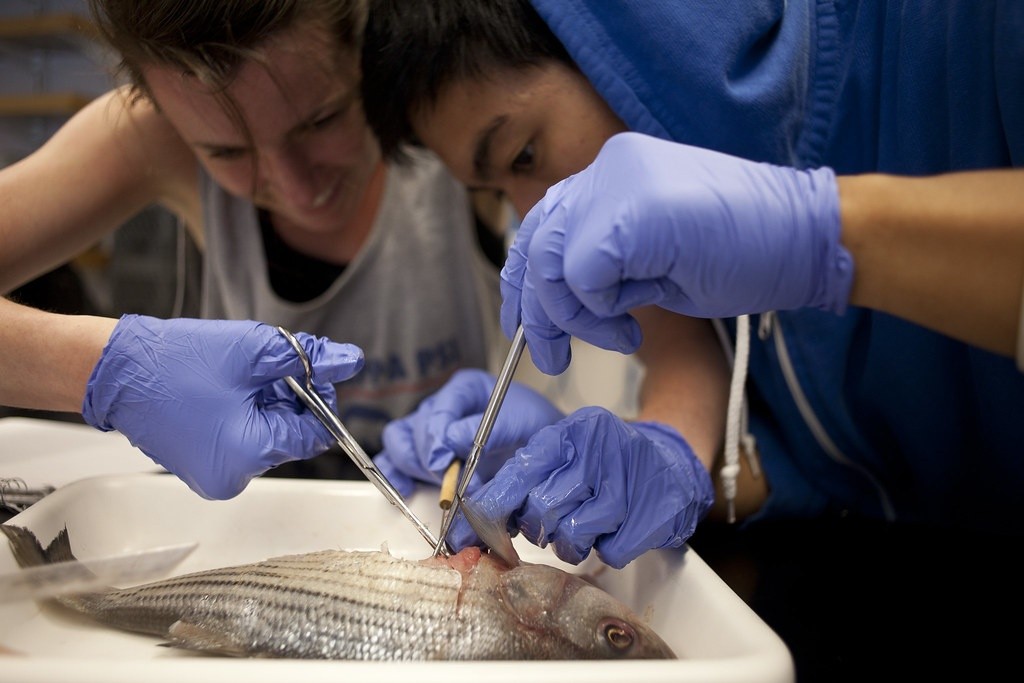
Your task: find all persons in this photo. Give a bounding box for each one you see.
[355,1,1024,683]
[0,1,729,565]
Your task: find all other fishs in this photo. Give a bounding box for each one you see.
[0,524,677,658]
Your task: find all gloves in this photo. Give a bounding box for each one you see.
[444,406,716,570]
[82,313,366,501]
[499,131,855,377]
[374,367,567,500]
